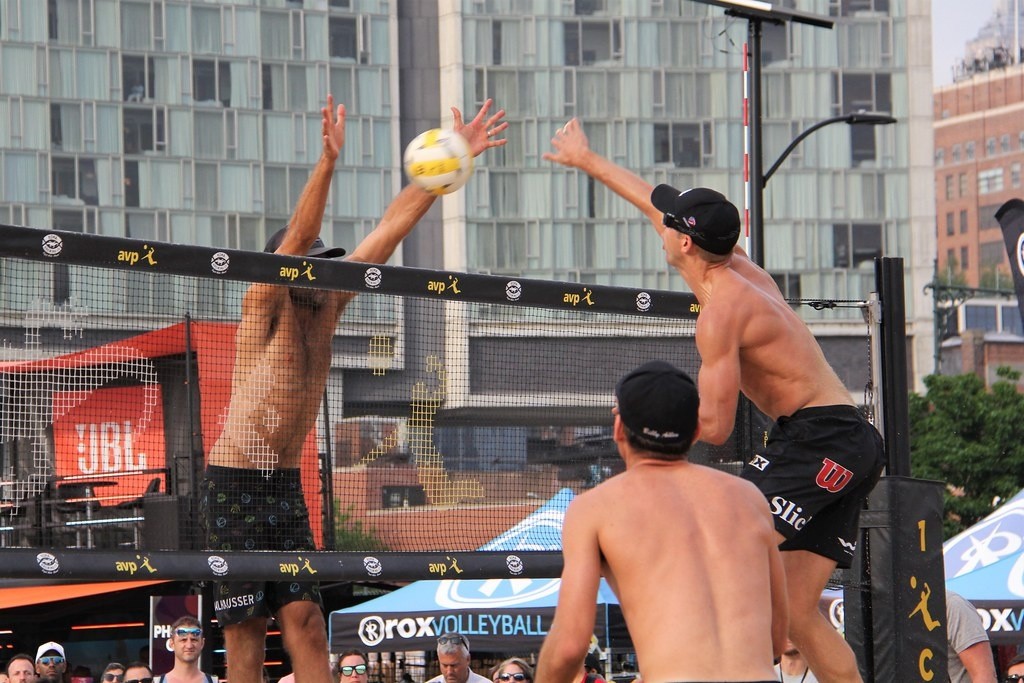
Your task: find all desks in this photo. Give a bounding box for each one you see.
[67,481,119,549]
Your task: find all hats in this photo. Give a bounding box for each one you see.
[263,223,346,257]
[615,361,700,444]
[651,184,741,256]
[35,641,65,663]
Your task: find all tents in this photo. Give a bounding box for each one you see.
[328,486,648,683]
[939,484,1024,683]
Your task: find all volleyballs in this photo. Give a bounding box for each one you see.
[402,128,474,194]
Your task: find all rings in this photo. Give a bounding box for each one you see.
[486,130,492,137]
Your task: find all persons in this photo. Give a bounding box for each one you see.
[200,94,517,683]
[0,609,645,683]
[539,119,887,683]
[539,361,790,683]
[942,589,1024,683]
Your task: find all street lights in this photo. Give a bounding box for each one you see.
[749,113,900,270]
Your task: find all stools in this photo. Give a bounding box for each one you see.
[117,473,163,548]
[55,485,99,548]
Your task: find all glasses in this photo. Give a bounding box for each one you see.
[339,664,367,676]
[437,636,469,651]
[501,673,525,681]
[125,678,152,683]
[172,627,201,637]
[663,212,689,234]
[38,656,64,665]
[104,673,122,682]
[1005,674,1024,683]
